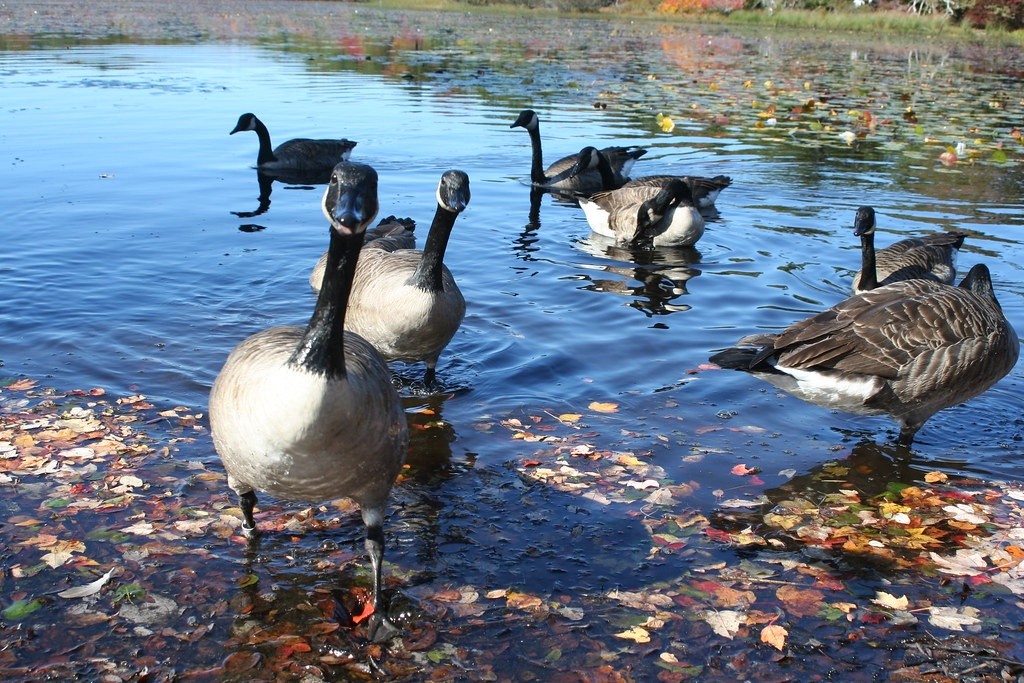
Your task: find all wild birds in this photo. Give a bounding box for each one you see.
[209,162,472,639]
[698,204,1020,463]
[230,114,360,183]
[508,108,734,248]
